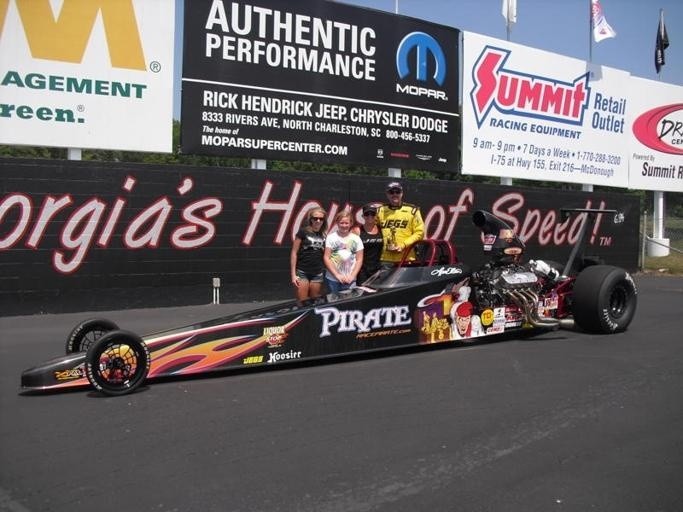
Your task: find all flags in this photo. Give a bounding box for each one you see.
[589,0,617,44]
[653,9,670,74]
[501,0,517,27]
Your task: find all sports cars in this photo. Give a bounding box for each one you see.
[15,205,639,395]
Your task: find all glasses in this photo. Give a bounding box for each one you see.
[387,189,404,195]
[311,216,325,222]
[362,212,377,217]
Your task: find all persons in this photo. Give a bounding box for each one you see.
[351,202,384,287]
[375,181,426,270]
[322,210,364,294]
[449,302,483,338]
[289,207,326,302]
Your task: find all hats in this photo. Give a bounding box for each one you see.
[387,182,404,192]
[362,202,377,214]
